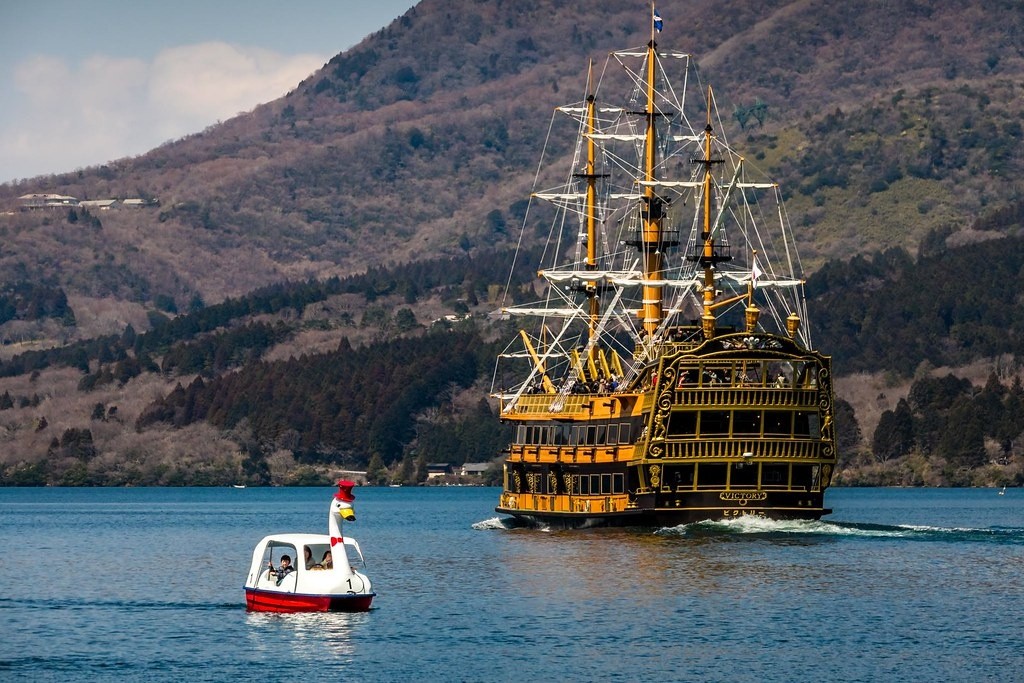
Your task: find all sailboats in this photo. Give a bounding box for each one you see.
[484,0,842,532]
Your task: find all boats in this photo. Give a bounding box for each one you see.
[389,484,400,488]
[233,484,246,489]
[243,480,377,614]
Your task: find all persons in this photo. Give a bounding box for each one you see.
[268,545,333,586]
[633,325,750,349]
[678,366,790,388]
[525,367,658,394]
